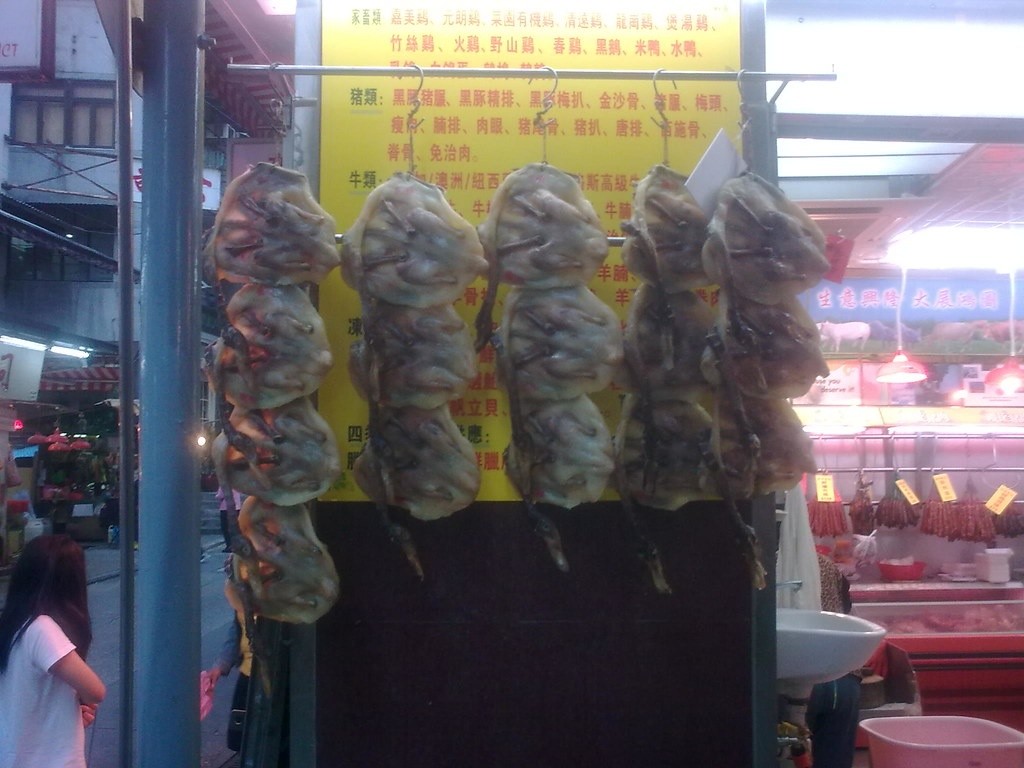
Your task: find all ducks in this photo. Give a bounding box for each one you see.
[202,161,831,697]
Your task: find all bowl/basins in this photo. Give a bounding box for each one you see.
[880,562,927,582]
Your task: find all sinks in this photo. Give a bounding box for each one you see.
[776,606,888,684]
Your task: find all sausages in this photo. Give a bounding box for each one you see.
[807,488,1024,543]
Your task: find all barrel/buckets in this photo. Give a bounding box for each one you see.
[107,525,116,544]
[24,518,44,543]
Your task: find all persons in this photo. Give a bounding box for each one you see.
[805,555,863,768]
[0,533,106,768]
[215,486,241,553]
[204,610,258,752]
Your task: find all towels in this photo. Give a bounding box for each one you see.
[778,482,822,609]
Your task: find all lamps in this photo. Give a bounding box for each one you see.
[69,436,92,449]
[44,424,68,443]
[48,441,68,451]
[27,408,47,443]
[986,274,1024,388]
[876,268,928,382]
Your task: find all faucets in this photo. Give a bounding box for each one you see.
[775,579,804,593]
